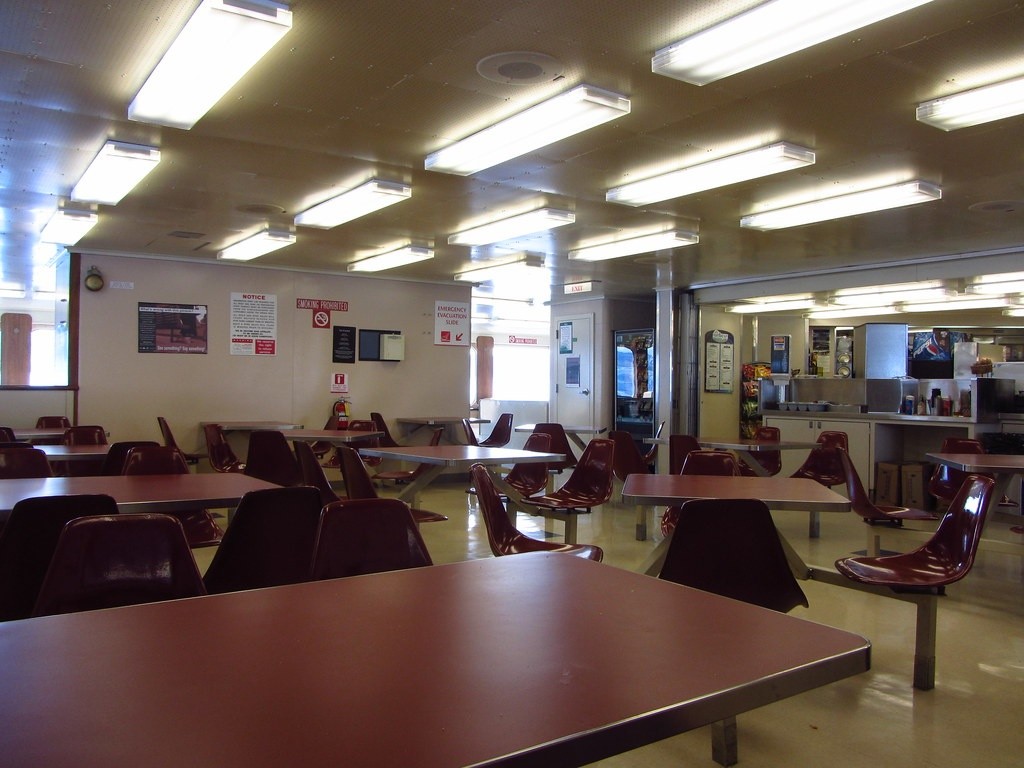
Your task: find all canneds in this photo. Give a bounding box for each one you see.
[912,334,944,359]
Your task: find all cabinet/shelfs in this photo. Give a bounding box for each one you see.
[765,418,872,500]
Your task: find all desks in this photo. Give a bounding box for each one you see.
[204,419,306,433]
[9,426,76,439]
[33,442,118,461]
[0,550,871,767]
[0,473,284,531]
[358,445,565,505]
[925,453,1024,473]
[273,428,386,442]
[619,473,852,581]
[398,417,492,448]
[515,424,608,451]
[646,438,824,475]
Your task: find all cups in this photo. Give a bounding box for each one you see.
[913,334,945,359]
[905,388,952,417]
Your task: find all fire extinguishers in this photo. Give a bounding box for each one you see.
[333,396,352,430]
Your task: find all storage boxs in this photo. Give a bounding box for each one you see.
[876,460,902,507]
[901,463,936,509]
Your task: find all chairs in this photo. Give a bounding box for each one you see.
[0,424,1024,768]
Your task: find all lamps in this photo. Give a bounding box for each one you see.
[39,0,1024,319]
